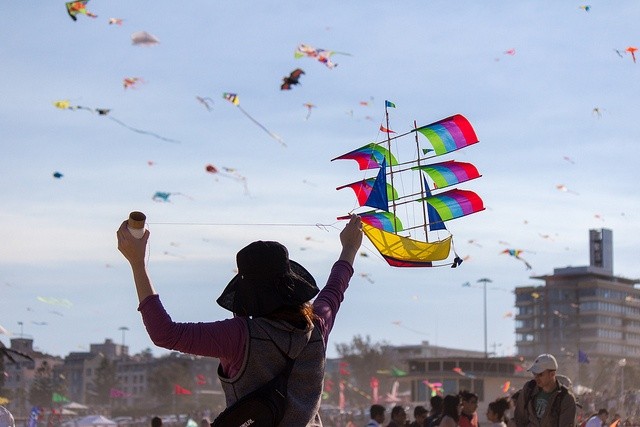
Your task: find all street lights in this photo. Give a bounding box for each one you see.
[119,327,128,360]
[18,321,22,337]
[477,278,491,355]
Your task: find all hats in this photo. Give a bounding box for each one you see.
[216,240,320,317]
[526,353,558,373]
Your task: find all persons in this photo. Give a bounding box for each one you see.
[366,404,386,427]
[408,405,429,427]
[199,417,210,427]
[438,395,461,426]
[386,406,410,425]
[514,354,576,426]
[151,417,162,427]
[583,409,608,427]
[458,391,479,427]
[115,214,365,427]
[511,389,522,421]
[486,398,510,426]
[424,396,442,427]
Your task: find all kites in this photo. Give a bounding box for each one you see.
[577,4,595,13]
[207,165,215,173]
[303,97,375,126]
[499,381,510,393]
[131,30,160,46]
[65,1,98,21]
[295,43,348,70]
[294,227,371,282]
[54,100,70,110]
[501,248,522,259]
[224,93,239,105]
[154,191,169,201]
[53,172,62,178]
[486,47,617,269]
[327,97,485,271]
[109,18,123,24]
[388,367,407,377]
[627,47,637,53]
[98,108,110,115]
[279,70,305,90]
[453,368,461,372]
[577,350,590,364]
[123,76,140,89]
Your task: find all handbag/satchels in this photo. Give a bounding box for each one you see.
[210,375,288,427]
